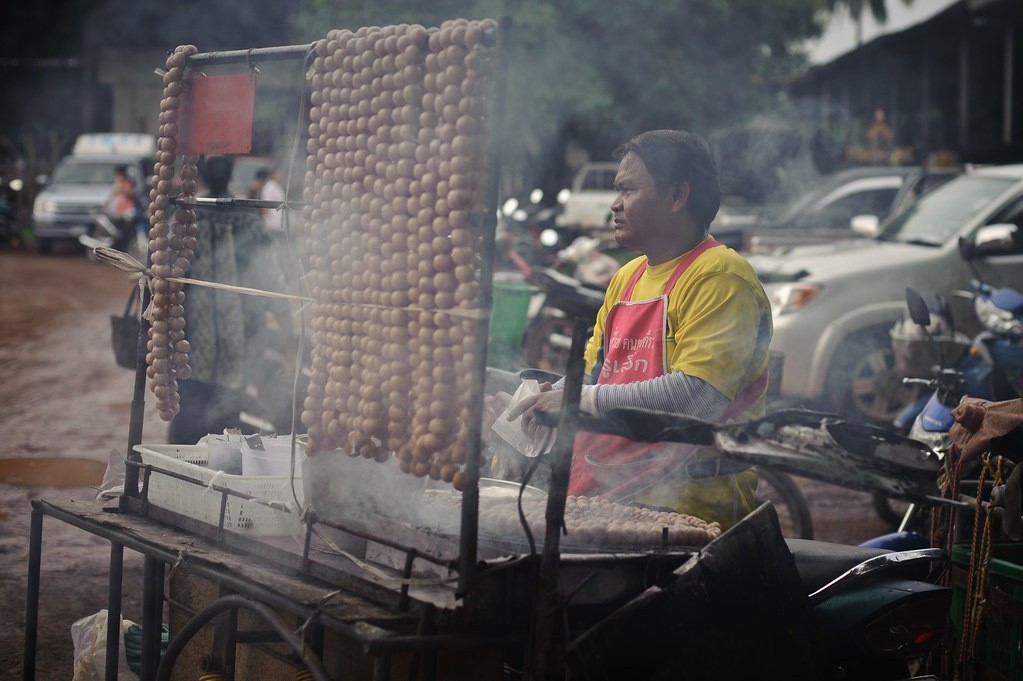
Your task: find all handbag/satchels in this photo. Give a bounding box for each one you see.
[110,282,143,369]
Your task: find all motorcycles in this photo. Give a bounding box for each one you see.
[25,405,1003,681]
[496,187,621,380]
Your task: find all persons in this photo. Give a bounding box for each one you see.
[99,163,138,255]
[139,143,296,451]
[247,168,286,235]
[507,128,773,534]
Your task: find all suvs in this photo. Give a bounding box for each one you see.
[34,154,155,252]
[752,162,1023,453]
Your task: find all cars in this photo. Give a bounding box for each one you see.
[708,166,952,262]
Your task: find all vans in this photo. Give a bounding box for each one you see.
[561,164,635,227]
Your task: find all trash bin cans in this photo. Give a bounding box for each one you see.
[489,277,544,370]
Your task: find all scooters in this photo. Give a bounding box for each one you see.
[875,240,1023,533]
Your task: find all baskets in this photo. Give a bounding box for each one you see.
[890,322,972,382]
[133,444,303,537]
